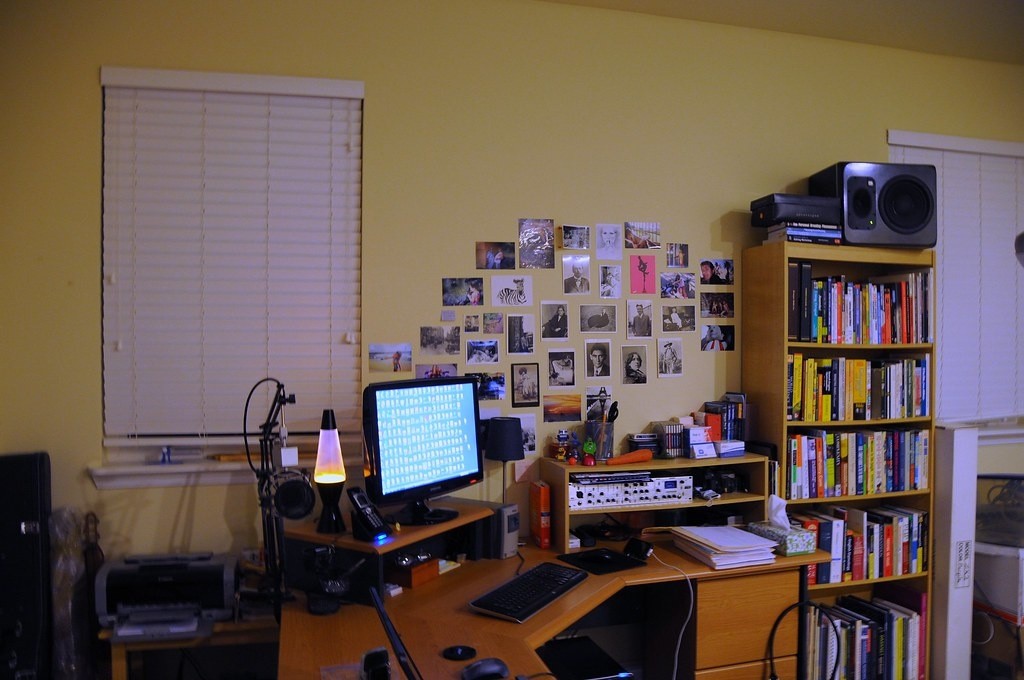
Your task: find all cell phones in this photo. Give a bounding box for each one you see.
[624,537,653,560]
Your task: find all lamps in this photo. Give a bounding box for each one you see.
[313,409,347,534]
[243,378,316,605]
[485,417,526,546]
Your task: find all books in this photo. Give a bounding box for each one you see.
[660,393,743,459]
[671,523,778,572]
[788,268,929,680]
[529,480,552,549]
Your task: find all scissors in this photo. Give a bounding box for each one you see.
[608,400,619,423]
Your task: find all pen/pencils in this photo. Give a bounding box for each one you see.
[599,409,607,458]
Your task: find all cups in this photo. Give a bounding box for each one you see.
[585,419,615,461]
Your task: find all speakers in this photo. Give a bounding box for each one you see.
[808,161,938,249]
[491,503,521,559]
[303,544,340,615]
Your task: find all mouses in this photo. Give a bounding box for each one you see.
[461,658,508,680]
[584,548,625,564]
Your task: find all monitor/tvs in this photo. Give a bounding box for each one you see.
[370,587,423,680]
[363,375,484,525]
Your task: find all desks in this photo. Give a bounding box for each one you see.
[99,614,278,680]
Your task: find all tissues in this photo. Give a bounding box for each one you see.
[747,495,816,556]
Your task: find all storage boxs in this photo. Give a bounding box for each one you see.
[932,425,978,680]
[971,475,1024,680]
[751,193,841,227]
[391,558,440,588]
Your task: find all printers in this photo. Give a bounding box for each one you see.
[95,550,241,641]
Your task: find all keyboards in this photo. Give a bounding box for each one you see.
[467,562,589,623]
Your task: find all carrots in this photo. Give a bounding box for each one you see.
[606,449,652,465]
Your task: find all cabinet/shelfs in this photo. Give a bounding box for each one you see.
[539,451,768,555]
[278,497,832,680]
[742,241,936,680]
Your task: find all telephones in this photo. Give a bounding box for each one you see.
[346,486,393,543]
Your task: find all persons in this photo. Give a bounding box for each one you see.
[700,260,724,285]
[565,263,589,294]
[668,305,683,329]
[467,279,481,305]
[589,343,609,377]
[661,341,679,375]
[622,352,646,384]
[631,304,652,336]
[541,305,567,338]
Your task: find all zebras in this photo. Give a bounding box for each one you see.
[495,278,527,305]
